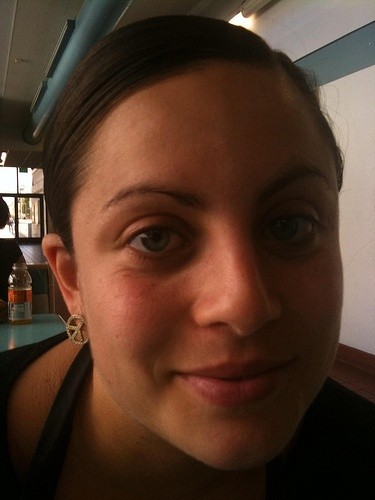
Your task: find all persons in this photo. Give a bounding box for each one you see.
[0,15,375,500]
[0,195,28,318]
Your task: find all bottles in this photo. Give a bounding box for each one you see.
[7,262,33,325]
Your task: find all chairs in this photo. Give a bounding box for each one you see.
[25,261,57,315]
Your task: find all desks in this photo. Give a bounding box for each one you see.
[1,313,72,351]
